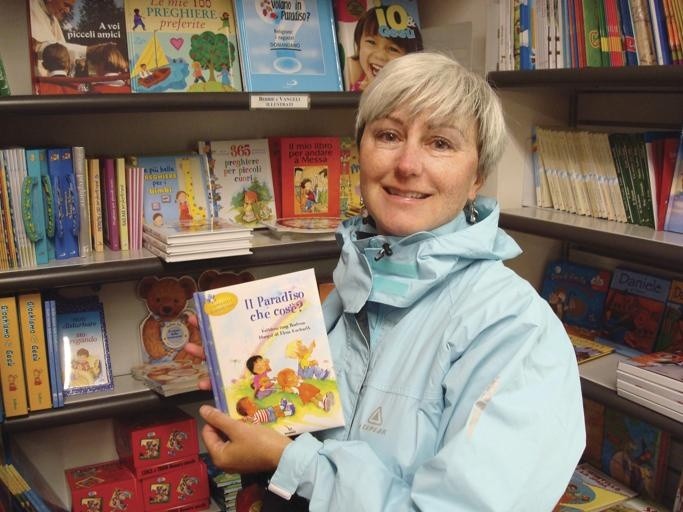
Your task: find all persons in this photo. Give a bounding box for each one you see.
[198,47,591,512]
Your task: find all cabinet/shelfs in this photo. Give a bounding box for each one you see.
[486,59,683,511]
[0,91,364,511]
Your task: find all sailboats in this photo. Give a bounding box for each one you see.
[129,29,172,90]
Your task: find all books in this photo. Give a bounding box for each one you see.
[0,1,425,97]
[495,1,683,71]
[0,137,366,272]
[555,406,682,511]
[522,125,682,234]
[540,257,682,423]
[0,267,344,511]
